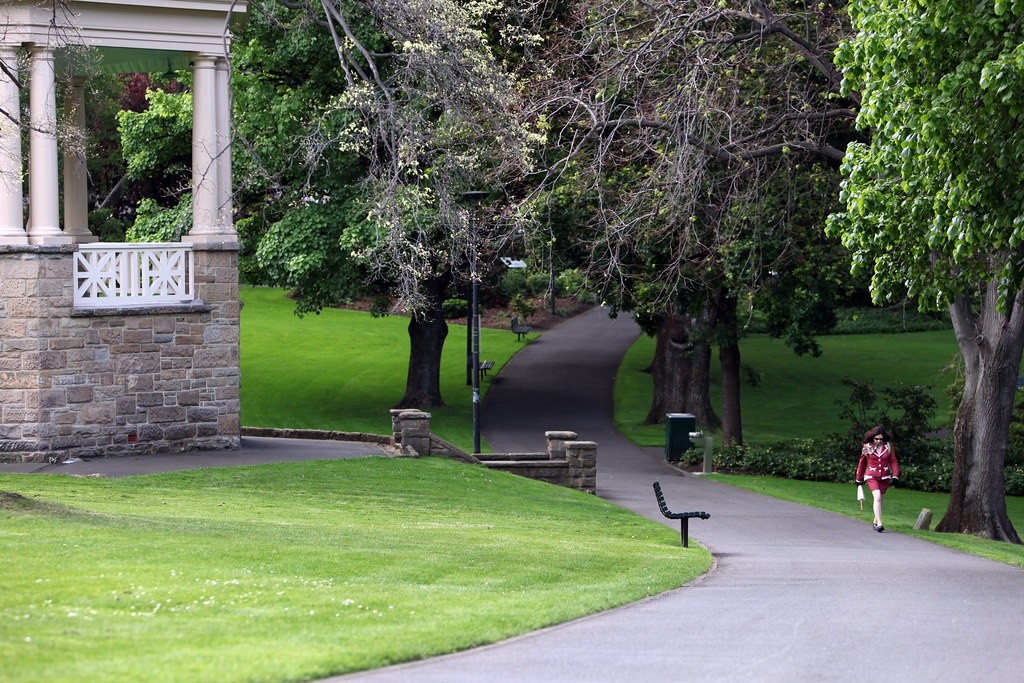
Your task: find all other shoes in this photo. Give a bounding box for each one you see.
[876,525,884,533]
[872,523,877,530]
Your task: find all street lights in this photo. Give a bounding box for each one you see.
[456,191,494,384]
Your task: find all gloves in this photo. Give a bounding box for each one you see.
[855,481,863,487]
[891,478,898,486]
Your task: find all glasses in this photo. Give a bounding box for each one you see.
[874,438,883,440]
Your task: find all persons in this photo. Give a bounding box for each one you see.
[855,425,899,532]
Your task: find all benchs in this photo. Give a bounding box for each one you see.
[478,360,495,381]
[511,314,531,342]
[653,481,710,548]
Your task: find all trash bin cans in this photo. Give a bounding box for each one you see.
[665,413,698,461]
